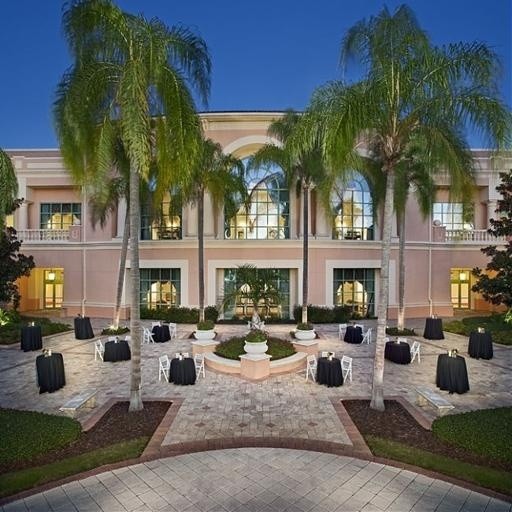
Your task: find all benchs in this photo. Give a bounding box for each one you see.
[58,387,100,419]
[414,384,456,418]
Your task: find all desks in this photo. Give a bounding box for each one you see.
[468,330,492,359]
[424,317,442,339]
[75,316,92,339]
[36,353,65,390]
[437,354,466,391]
[21,325,42,351]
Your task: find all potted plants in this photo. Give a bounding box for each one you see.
[243,328,269,357]
[196,318,217,342]
[294,322,317,341]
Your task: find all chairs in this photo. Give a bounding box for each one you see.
[94,319,207,385]
[305,322,421,387]
